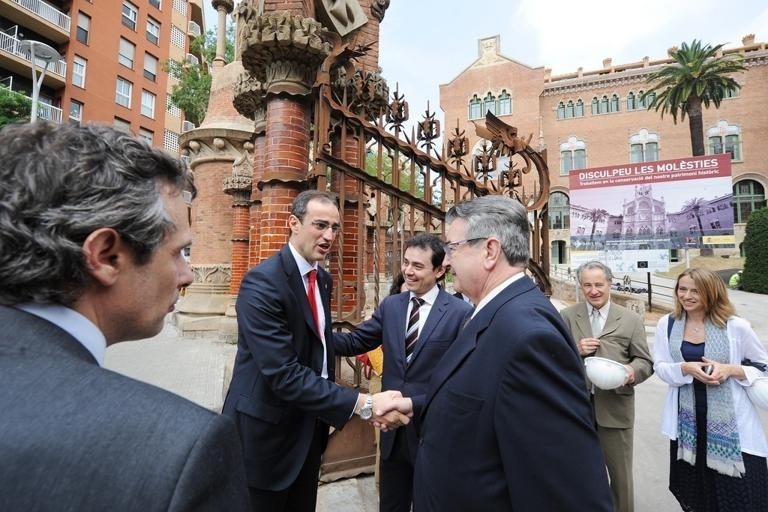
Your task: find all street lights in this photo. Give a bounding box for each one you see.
[17,39,62,127]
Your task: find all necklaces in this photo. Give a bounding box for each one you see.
[687,318,704,332]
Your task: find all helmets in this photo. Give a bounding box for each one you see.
[584,356,629,390]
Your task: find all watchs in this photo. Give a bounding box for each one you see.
[357,390,375,424]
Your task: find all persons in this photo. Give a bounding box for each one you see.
[329,233,478,511]
[221,189,410,511]
[0,120,257,511]
[654,266,767,512]
[365,195,614,509]
[367,271,408,497]
[728,270,744,290]
[559,262,655,512]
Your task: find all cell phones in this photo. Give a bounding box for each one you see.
[706,365,713,374]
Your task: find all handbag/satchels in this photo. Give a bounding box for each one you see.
[355,352,374,380]
[742,376,768,410]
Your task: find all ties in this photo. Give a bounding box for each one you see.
[307,269,322,339]
[592,308,602,338]
[405,297,426,366]
